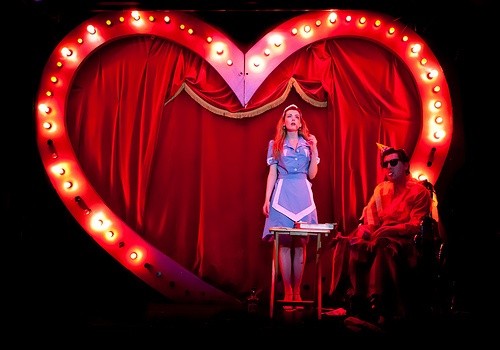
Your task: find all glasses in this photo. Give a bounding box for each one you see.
[380,157,405,169]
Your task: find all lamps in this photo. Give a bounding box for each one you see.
[36,8,447,318]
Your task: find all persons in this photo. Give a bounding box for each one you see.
[340,142,435,323]
[262,103,321,311]
